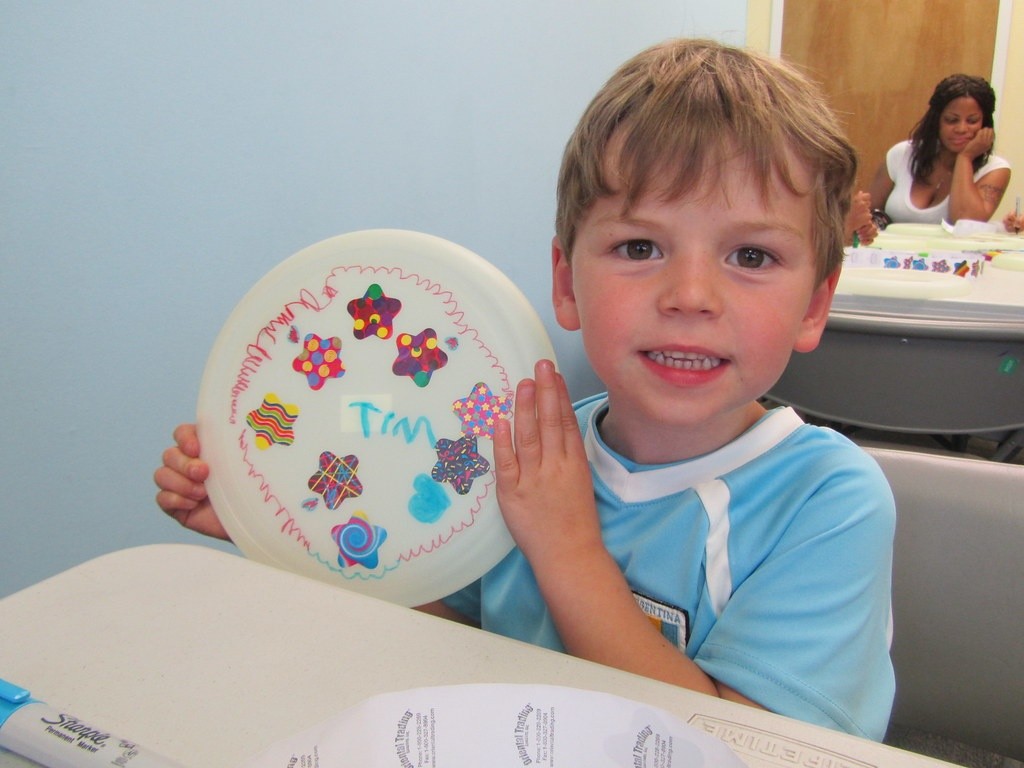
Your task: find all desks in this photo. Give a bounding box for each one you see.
[0,543,961,768]
[762,223,1023,461]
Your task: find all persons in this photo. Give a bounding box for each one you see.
[832,73,1024,251]
[156,40,894,743]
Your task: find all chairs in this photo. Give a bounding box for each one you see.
[761,308,1024,759]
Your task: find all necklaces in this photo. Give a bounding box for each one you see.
[929,165,954,190]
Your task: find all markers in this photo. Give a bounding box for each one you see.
[853,230,859,248]
[1015,195,1022,237]
[1,678,182,768]
[865,245,929,257]
